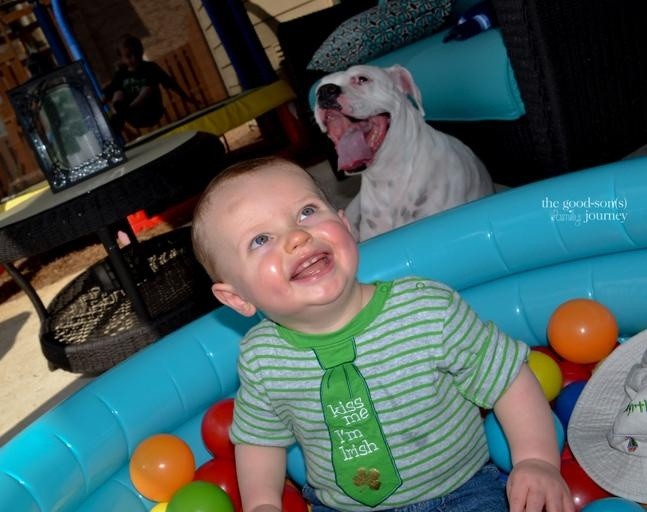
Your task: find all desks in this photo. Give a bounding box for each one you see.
[1,77,298,375]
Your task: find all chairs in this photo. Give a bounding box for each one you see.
[275,0,647,188]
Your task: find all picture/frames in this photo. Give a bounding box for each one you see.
[5,59,127,195]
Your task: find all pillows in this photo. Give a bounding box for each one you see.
[307,0,452,73]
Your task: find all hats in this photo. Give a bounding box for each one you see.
[567,328,647,505]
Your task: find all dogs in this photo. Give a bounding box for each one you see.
[311,62,496,243]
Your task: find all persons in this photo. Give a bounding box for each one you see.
[191,155,576,512]
